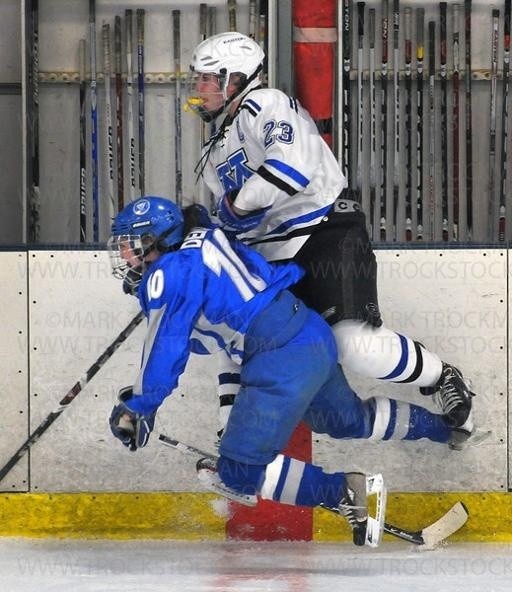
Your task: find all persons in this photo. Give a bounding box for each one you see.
[108,196,474,547]
[190,31,471,477]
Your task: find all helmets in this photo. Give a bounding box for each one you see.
[190,31,265,79]
[110,196,186,248]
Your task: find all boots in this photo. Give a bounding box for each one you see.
[332,471,368,546]
[419,360,474,451]
[195,457,220,474]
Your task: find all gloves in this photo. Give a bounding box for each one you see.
[188,190,271,238]
[108,385,154,451]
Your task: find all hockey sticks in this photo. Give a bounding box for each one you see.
[150,430,468,544]
